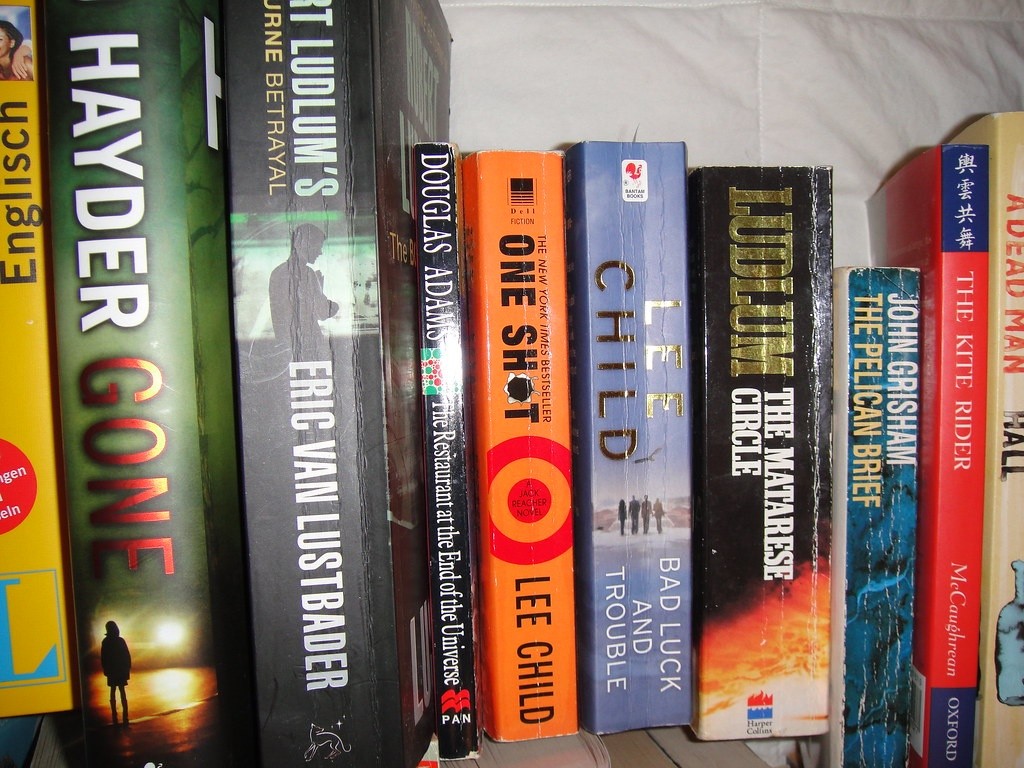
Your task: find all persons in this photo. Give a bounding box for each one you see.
[101,621,131,728]
[0,19,33,81]
[618,495,666,535]
[269,224,339,338]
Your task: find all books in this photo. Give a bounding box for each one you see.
[1,1,1023,767]
